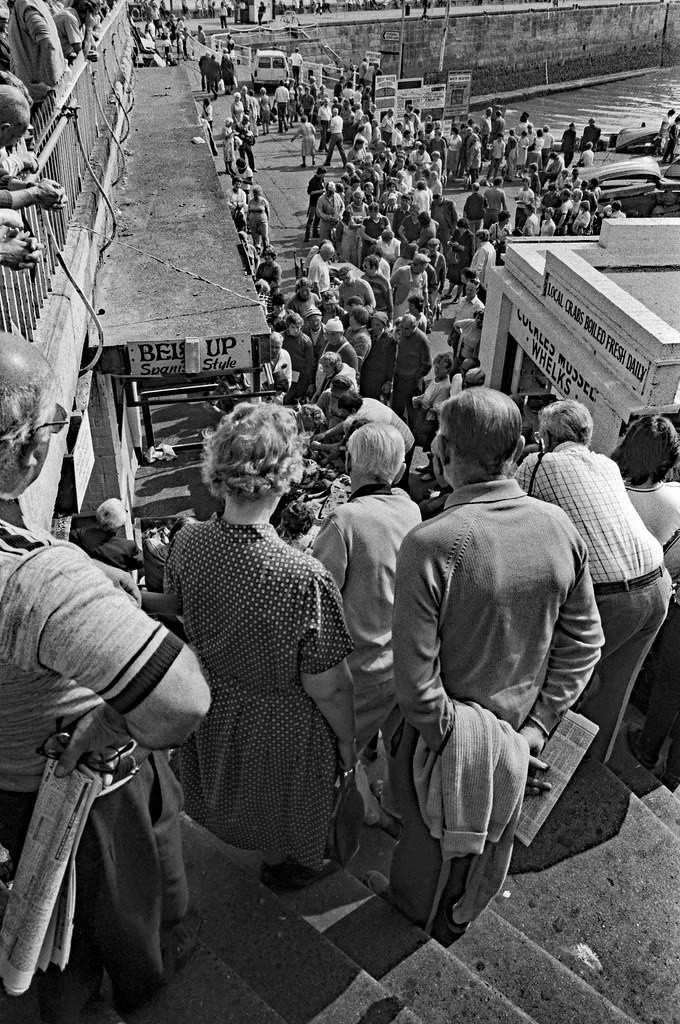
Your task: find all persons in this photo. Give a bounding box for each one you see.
[0,332,211,1024]
[0,0,680,933]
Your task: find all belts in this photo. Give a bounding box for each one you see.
[593,561,666,593]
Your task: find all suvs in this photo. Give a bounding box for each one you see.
[250,48,290,94]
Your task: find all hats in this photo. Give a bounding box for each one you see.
[324,316,344,333]
[271,294,286,307]
[427,238,440,245]
[307,305,322,318]
[371,311,388,325]
[331,375,353,390]
[337,266,351,276]
[413,253,431,265]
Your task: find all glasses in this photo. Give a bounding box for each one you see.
[534,432,547,442]
[21,403,68,454]
[35,716,134,773]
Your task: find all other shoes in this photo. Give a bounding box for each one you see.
[370,778,404,840]
[452,298,459,303]
[361,869,431,933]
[283,839,360,887]
[441,294,452,300]
[112,913,210,1009]
[415,465,433,472]
[442,897,471,933]
[420,472,434,480]
